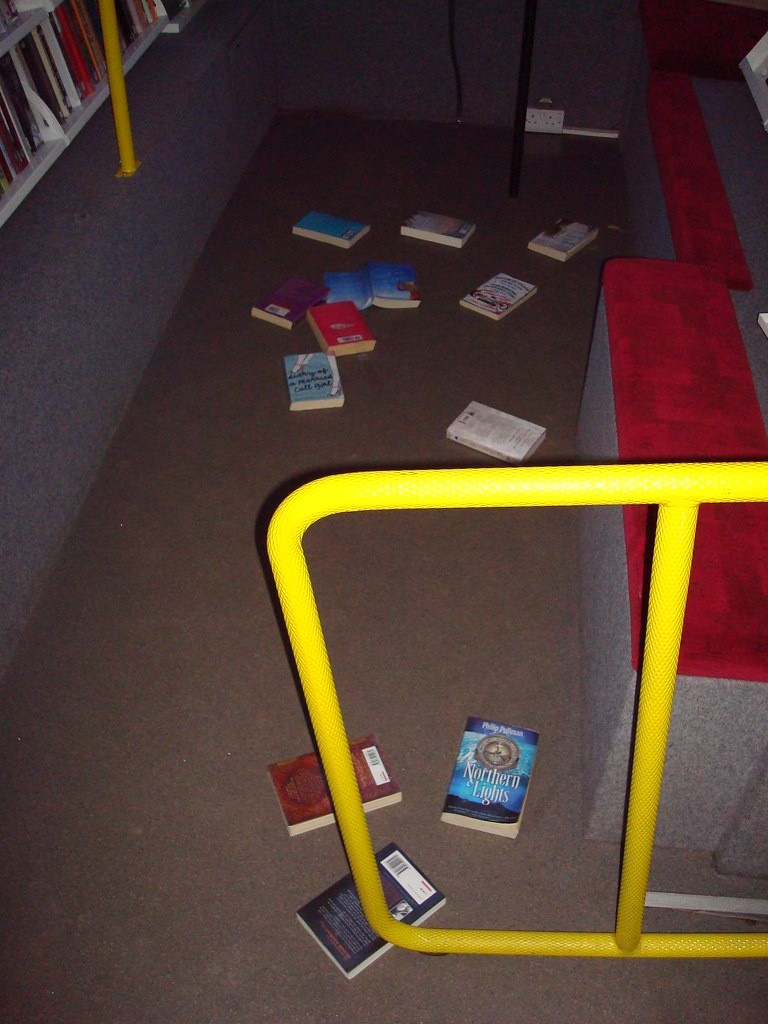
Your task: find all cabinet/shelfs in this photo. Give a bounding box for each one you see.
[0,0,206,230]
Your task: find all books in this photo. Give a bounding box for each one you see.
[324,260,423,312]
[526,216,599,264]
[294,842,446,980]
[400,210,476,249]
[0,1,158,199]
[267,734,403,838]
[459,272,538,320]
[440,715,541,840]
[251,274,331,330]
[304,300,377,356]
[291,210,371,249]
[445,400,547,466]
[284,351,344,412]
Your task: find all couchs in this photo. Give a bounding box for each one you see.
[573,0,768,849]
